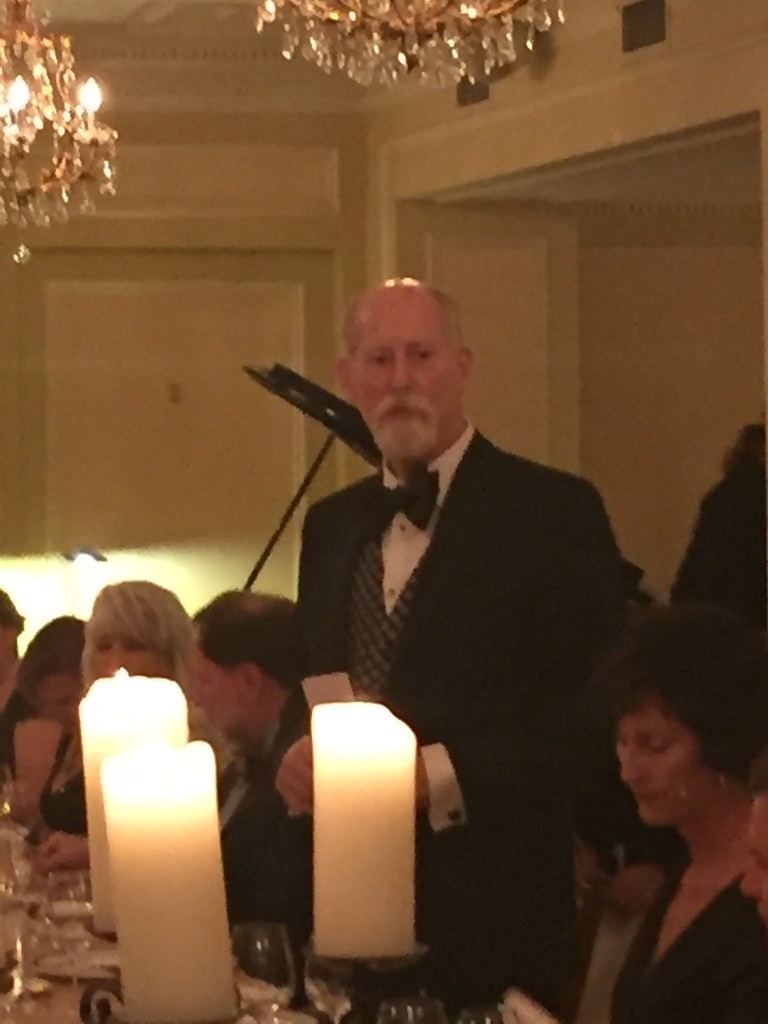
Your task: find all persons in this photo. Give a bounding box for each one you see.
[264,277,624,1024]
[511,601,766,1024]
[667,420,766,696]
[22,577,226,850]
[569,728,689,1021]
[1,585,93,806]
[38,589,320,1008]
[483,761,768,1021]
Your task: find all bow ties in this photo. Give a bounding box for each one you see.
[371,470,438,537]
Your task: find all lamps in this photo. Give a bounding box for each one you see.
[1,1,120,266]
[255,1,566,88]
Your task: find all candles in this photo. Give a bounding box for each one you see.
[77,664,190,934]
[100,741,238,1024]
[311,699,422,961]
